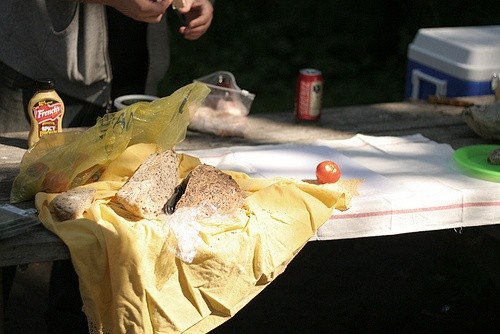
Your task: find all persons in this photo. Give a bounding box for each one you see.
[0,0,216,132]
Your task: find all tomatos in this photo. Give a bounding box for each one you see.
[315,160,341,184]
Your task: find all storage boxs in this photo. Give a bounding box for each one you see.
[406,25,499,103]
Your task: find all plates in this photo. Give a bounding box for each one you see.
[453,144,500,180]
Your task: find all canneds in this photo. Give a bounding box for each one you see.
[295,68,325,122]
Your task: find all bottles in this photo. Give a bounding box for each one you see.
[28,77,66,153]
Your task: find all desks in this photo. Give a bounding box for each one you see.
[0,92,496,334]
